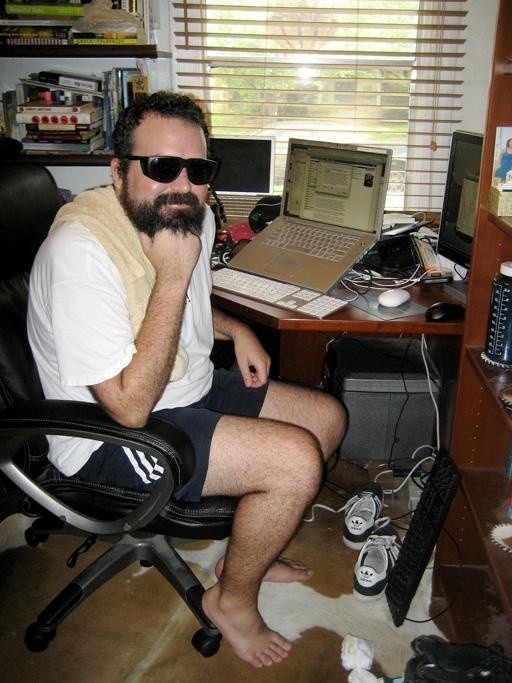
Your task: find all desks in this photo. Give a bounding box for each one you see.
[208,241,470,387]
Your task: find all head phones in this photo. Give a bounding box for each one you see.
[205,180,227,228]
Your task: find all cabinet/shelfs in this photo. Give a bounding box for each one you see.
[0,44,158,166]
[430,0,512,665]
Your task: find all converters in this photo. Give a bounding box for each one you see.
[393,458,422,478]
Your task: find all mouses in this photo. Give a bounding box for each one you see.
[378,288,411,308]
[425,301,465,322]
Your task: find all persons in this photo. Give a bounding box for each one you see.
[26,90,350,669]
[494,138,512,183]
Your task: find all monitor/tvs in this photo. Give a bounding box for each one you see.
[436,129,483,268]
[206,135,277,196]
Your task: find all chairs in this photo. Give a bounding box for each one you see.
[0,131,241,658]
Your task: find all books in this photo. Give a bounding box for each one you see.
[0,1,150,153]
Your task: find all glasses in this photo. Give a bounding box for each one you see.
[116,156,218,185]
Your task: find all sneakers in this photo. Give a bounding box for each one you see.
[342,482,384,550]
[352,528,402,601]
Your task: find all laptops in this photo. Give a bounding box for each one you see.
[228,139,391,294]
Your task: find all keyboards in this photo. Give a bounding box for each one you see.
[384,444,464,628]
[211,268,349,320]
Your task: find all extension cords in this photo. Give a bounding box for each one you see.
[407,461,432,509]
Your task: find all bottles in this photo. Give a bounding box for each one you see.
[484,262,512,365]
